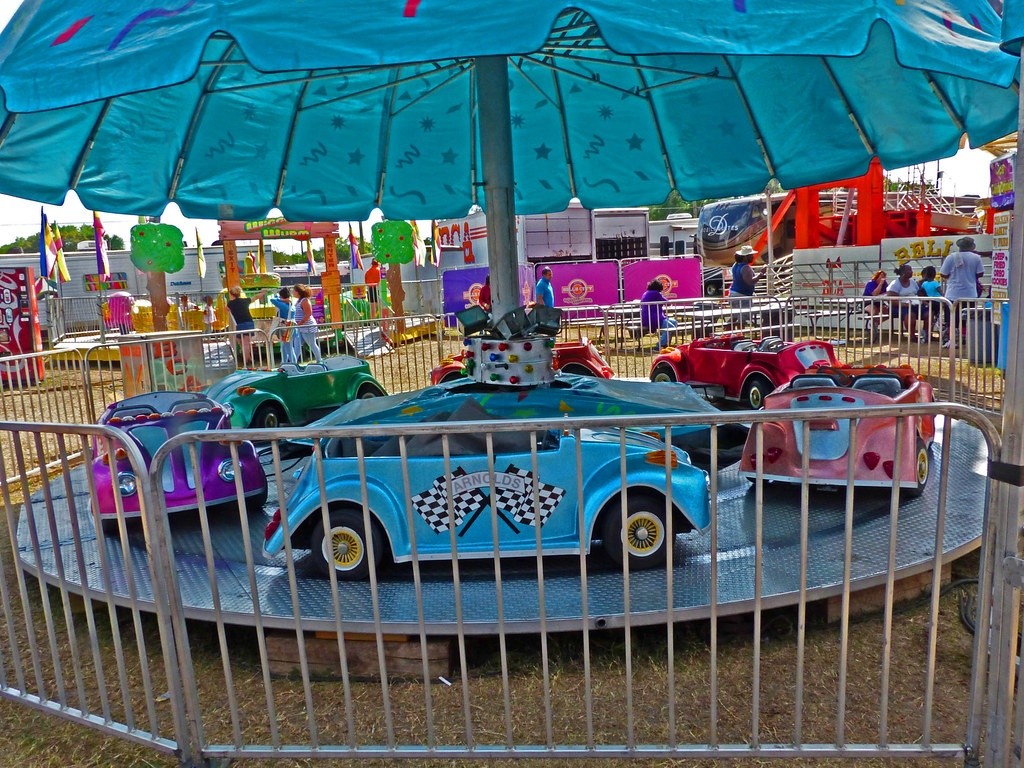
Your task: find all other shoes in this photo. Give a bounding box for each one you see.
[941,337,950,348]
[653,345,663,353]
[909,335,918,344]
[869,322,879,335]
[921,329,928,340]
[920,337,927,344]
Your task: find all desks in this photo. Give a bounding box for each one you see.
[674,308,790,332]
[556,301,635,328]
[820,299,874,339]
[602,306,692,332]
[694,297,809,339]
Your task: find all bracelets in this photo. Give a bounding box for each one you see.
[760,272,763,275]
[484,303,487,307]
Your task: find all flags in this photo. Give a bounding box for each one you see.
[411,219,426,267]
[93,212,110,280]
[306,240,315,272]
[39,214,72,284]
[348,226,364,270]
[431,220,442,266]
[196,229,206,278]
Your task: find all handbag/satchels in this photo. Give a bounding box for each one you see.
[267,316,297,342]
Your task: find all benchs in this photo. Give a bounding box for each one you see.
[325,356,362,370]
[113,389,201,413]
[104,397,215,422]
[559,307,933,352]
[790,372,904,398]
[275,364,325,377]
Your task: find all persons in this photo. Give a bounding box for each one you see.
[226,286,265,365]
[639,279,677,352]
[365,258,380,319]
[724,245,766,332]
[860,264,945,341]
[479,273,493,313]
[203,296,217,338]
[293,283,321,362]
[270,287,297,363]
[535,266,556,343]
[938,237,985,349]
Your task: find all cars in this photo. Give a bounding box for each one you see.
[87,391,268,536]
[738,364,937,499]
[430,336,615,385]
[192,356,389,428]
[649,332,842,410]
[263,397,711,582]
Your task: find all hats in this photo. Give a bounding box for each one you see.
[735,246,758,256]
